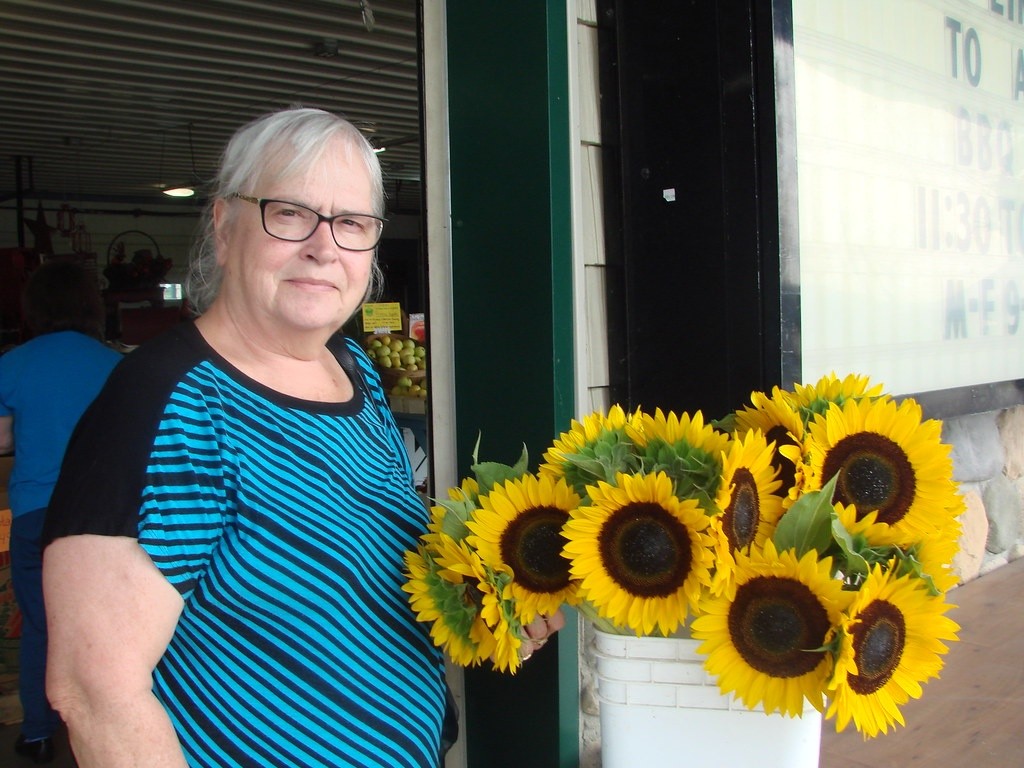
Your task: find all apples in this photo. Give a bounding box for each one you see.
[366,336,427,399]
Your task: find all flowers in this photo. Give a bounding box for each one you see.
[402,376,966,742]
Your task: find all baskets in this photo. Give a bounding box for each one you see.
[362,332,426,389]
[103,230,173,288]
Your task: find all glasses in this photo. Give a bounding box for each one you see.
[224,191,389,251]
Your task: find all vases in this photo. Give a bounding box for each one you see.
[589,623,825,768]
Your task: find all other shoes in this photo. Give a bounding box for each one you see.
[15,734,54,765]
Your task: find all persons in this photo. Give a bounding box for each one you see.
[0,258,126,765]
[41,109,564,768]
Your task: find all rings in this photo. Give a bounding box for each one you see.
[522,654,532,661]
